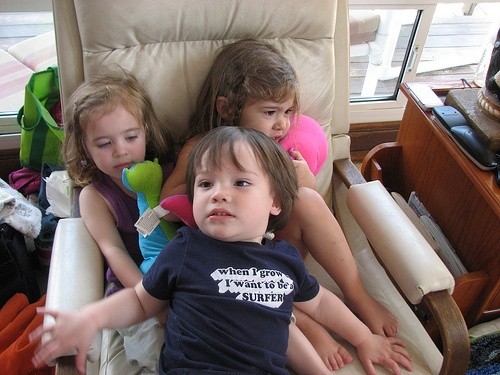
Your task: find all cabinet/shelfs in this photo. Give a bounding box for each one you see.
[361,80,500,328]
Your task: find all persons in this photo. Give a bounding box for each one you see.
[63,39,400,375]
[29,124,413,375]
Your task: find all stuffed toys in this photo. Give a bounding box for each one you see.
[160,115,328,234]
[121,157,181,273]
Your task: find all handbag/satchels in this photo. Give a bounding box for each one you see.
[16,67,68,172]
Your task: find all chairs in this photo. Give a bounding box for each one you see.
[40,0,471,375]
